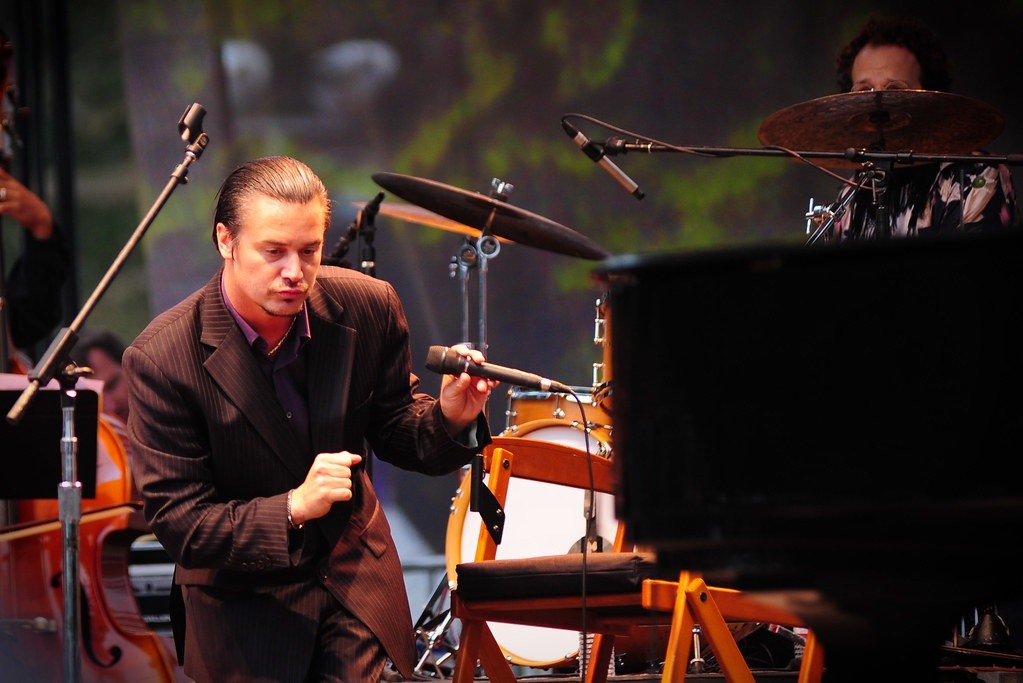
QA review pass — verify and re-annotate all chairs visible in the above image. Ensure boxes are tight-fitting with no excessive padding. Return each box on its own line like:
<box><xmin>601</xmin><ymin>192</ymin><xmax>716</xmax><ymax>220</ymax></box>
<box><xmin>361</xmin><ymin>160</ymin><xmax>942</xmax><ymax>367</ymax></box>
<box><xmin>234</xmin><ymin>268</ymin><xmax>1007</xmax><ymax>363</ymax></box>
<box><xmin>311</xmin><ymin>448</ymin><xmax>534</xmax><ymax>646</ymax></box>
<box><xmin>448</xmin><ymin>437</ymin><xmax>825</xmax><ymax>683</ymax></box>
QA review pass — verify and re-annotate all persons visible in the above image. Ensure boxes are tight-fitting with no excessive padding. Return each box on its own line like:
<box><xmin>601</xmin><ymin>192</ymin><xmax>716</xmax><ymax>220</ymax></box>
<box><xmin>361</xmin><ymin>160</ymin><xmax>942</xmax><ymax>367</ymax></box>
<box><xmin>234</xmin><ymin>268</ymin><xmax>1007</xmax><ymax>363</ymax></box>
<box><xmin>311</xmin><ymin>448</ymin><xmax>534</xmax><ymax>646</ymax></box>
<box><xmin>78</xmin><ymin>331</ymin><xmax>127</xmax><ymax>421</ymax></box>
<box><xmin>122</xmin><ymin>155</ymin><xmax>501</xmax><ymax>683</ymax></box>
<box><xmin>1</xmin><ymin>43</ymin><xmax>57</xmax><ymax>375</ymax></box>
<box><xmin>820</xmin><ymin>27</ymin><xmax>1015</xmax><ymax>245</ymax></box>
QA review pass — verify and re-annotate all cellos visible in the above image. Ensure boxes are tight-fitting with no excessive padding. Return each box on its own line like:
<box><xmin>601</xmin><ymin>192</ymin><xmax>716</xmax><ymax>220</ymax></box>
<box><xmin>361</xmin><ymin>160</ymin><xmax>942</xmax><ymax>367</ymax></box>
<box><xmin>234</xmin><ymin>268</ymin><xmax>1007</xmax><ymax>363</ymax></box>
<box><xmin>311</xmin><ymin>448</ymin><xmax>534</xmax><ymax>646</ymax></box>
<box><xmin>0</xmin><ymin>210</ymin><xmax>191</xmax><ymax>683</ymax></box>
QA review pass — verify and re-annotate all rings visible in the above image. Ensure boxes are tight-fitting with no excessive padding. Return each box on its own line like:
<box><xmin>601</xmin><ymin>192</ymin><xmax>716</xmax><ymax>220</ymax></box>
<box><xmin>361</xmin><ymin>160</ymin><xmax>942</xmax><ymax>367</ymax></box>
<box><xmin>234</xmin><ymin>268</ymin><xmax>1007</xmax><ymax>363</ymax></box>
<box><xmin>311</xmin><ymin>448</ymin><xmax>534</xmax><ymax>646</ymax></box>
<box><xmin>1</xmin><ymin>188</ymin><xmax>8</xmax><ymax>200</ymax></box>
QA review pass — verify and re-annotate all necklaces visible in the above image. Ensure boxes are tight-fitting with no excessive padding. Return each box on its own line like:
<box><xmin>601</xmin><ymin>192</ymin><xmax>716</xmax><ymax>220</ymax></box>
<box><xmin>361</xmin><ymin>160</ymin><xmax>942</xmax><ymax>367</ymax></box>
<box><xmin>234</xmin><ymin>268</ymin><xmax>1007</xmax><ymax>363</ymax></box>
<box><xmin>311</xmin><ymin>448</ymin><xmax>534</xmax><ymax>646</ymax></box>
<box><xmin>267</xmin><ymin>316</ymin><xmax>296</xmax><ymax>356</ymax></box>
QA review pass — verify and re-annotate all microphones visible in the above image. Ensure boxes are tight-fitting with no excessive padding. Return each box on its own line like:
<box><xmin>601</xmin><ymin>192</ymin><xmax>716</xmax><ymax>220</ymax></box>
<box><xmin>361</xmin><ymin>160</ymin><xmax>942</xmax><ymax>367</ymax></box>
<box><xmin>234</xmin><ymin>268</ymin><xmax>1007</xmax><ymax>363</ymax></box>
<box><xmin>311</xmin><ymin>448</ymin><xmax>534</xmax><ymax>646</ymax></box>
<box><xmin>326</xmin><ymin>192</ymin><xmax>386</xmax><ymax>266</ymax></box>
<box><xmin>561</xmin><ymin>119</ymin><xmax>644</xmax><ymax>200</ymax></box>
<box><xmin>425</xmin><ymin>345</ymin><xmax>572</xmax><ymax>394</ymax></box>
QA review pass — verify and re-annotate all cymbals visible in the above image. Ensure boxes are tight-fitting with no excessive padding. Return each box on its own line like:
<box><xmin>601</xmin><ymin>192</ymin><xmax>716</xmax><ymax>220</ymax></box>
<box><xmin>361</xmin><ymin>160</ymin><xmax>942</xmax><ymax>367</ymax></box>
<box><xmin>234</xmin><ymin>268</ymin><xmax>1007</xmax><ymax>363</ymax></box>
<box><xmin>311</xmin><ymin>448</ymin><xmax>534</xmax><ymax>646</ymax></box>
<box><xmin>353</xmin><ymin>198</ymin><xmax>514</xmax><ymax>247</ymax></box>
<box><xmin>370</xmin><ymin>169</ymin><xmax>610</xmax><ymax>264</ymax></box>
<box><xmin>755</xmin><ymin>88</ymin><xmax>1006</xmax><ymax>175</ymax></box>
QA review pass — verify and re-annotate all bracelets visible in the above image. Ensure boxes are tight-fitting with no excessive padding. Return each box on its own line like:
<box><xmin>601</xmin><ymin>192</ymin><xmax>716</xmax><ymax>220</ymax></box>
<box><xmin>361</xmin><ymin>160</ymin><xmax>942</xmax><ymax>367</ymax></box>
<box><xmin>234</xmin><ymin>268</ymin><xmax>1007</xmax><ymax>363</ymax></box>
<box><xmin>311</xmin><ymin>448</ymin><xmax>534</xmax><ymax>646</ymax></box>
<box><xmin>287</xmin><ymin>489</ymin><xmax>305</xmax><ymax>530</ymax></box>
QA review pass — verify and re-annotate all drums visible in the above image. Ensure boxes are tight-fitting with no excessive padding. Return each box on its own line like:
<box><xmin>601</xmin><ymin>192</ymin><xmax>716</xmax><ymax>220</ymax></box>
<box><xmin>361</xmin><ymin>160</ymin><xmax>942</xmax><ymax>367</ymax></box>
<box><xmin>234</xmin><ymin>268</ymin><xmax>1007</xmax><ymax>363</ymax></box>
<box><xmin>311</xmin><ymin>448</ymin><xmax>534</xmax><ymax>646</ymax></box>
<box><xmin>440</xmin><ymin>417</ymin><xmax>634</xmax><ymax>671</ymax></box>
<box><xmin>501</xmin><ymin>386</ymin><xmax>613</xmax><ymax>439</ymax></box>
